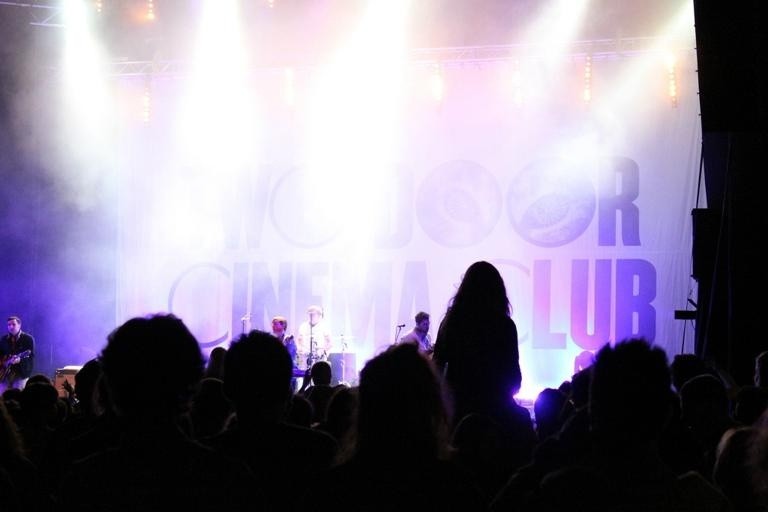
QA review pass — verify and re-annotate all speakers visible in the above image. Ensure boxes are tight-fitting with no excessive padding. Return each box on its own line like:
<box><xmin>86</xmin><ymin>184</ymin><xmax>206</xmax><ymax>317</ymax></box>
<box><xmin>54</xmin><ymin>369</ymin><xmax>78</xmax><ymax>398</ymax></box>
<box><xmin>327</xmin><ymin>353</ymin><xmax>357</xmax><ymax>385</ymax></box>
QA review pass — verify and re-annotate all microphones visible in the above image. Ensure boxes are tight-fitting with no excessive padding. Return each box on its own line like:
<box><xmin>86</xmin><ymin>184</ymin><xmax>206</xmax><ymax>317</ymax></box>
<box><xmin>397</xmin><ymin>324</ymin><xmax>405</xmax><ymax>327</ymax></box>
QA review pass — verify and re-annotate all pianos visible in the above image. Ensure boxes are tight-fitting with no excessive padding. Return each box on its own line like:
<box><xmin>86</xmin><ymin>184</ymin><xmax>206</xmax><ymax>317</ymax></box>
<box><xmin>291</xmin><ymin>367</ymin><xmax>311</xmax><ymax>378</ymax></box>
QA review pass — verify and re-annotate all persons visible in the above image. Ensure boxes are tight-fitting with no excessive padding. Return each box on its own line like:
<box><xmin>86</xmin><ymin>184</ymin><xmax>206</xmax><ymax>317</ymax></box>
<box><xmin>433</xmin><ymin>261</ymin><xmax>522</xmax><ymax>417</ymax></box>
<box><xmin>0</xmin><ymin>312</ymin><xmax>767</xmax><ymax>512</ymax></box>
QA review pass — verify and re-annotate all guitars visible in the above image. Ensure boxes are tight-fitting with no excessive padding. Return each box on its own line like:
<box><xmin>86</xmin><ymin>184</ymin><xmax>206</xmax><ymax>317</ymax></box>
<box><xmin>0</xmin><ymin>349</ymin><xmax>31</xmax><ymax>381</ymax></box>
